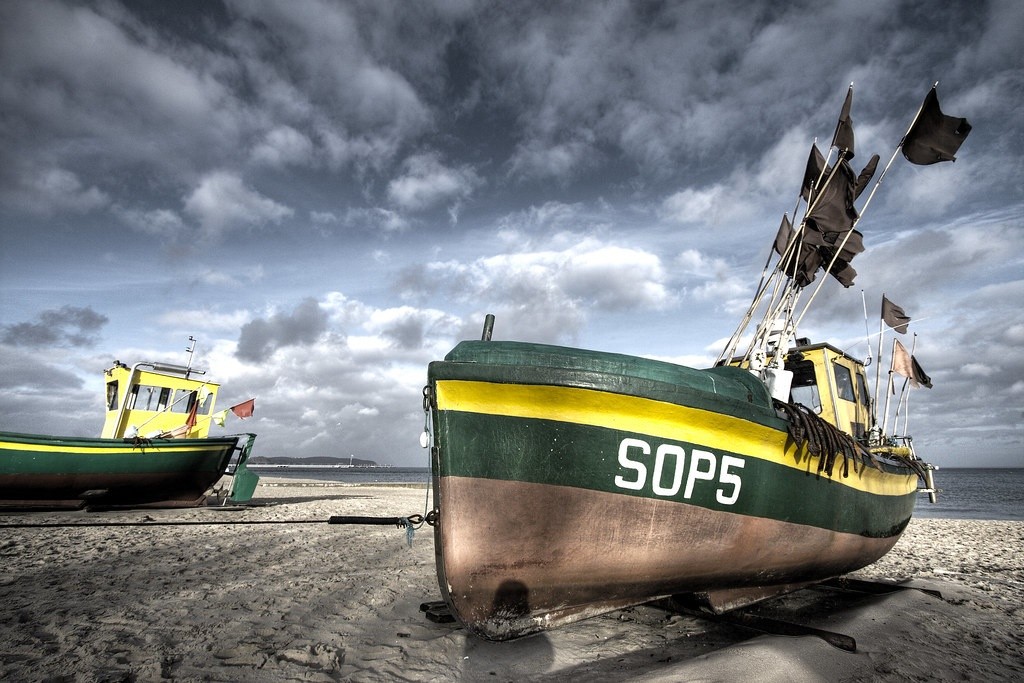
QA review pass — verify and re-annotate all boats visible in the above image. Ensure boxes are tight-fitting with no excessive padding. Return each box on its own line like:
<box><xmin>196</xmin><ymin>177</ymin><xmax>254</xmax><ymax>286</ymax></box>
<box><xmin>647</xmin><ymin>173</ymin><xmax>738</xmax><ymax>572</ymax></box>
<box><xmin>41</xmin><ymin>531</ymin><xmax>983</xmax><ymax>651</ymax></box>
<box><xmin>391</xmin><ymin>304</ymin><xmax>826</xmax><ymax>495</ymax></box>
<box><xmin>422</xmin><ymin>290</ymin><xmax>939</xmax><ymax>643</ymax></box>
<box><xmin>0</xmin><ymin>336</ymin><xmax>260</xmax><ymax>511</ymax></box>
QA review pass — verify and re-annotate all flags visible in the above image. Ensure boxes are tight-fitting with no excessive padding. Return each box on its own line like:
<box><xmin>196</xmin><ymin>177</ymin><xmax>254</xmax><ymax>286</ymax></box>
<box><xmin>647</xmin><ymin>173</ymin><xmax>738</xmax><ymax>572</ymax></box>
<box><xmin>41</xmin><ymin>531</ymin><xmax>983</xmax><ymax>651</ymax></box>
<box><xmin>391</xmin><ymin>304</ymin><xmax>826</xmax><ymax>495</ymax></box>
<box><xmin>898</xmin><ymin>87</ymin><xmax>973</xmax><ymax>165</ymax></box>
<box><xmin>774</xmin><ymin>215</ymin><xmax>796</xmax><ymax>257</ymax></box>
<box><xmin>911</xmin><ymin>355</ymin><xmax>932</xmax><ymax>388</ymax></box>
<box><xmin>881</xmin><ymin>298</ymin><xmax>911</xmax><ymax>334</ymax></box>
<box><xmin>196</xmin><ymin>385</ymin><xmax>210</xmax><ymax>407</ymax></box>
<box><xmin>212</xmin><ymin>410</ymin><xmax>230</xmax><ymax>427</ymax></box>
<box><xmin>780</xmin><ymin>88</ymin><xmax>880</xmax><ymax>288</ymax></box>
<box><xmin>231</xmin><ymin>398</ymin><xmax>254</xmax><ymax>418</ymax></box>
<box><xmin>185</xmin><ymin>399</ymin><xmax>199</xmax><ymax>433</ymax></box>
<box><xmin>892</xmin><ymin>340</ymin><xmax>919</xmax><ymax>389</ymax></box>
